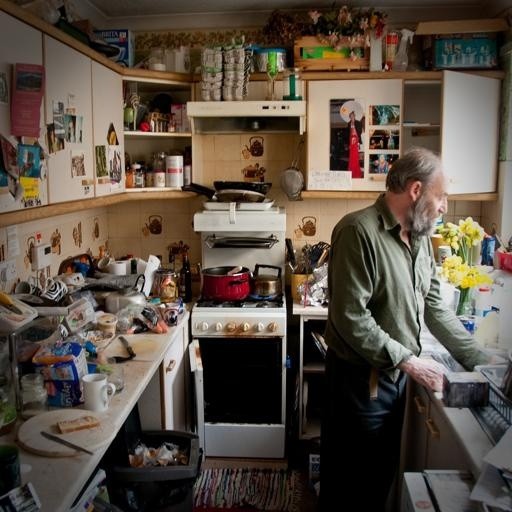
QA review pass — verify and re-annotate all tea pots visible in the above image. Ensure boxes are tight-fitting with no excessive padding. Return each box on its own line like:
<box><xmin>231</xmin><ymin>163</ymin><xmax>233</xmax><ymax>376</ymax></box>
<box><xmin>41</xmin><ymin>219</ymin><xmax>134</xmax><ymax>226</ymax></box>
<box><xmin>24</xmin><ymin>234</ymin><xmax>38</xmax><ymax>262</ymax></box>
<box><xmin>92</xmin><ymin>217</ymin><xmax>100</xmax><ymax>238</ymax></box>
<box><xmin>297</xmin><ymin>215</ymin><xmax>317</xmax><ymax>237</ymax></box>
<box><xmin>144</xmin><ymin>214</ymin><xmax>164</xmax><ymax>235</ymax></box>
<box><xmin>245</xmin><ymin>135</ymin><xmax>265</xmax><ymax>157</ymax></box>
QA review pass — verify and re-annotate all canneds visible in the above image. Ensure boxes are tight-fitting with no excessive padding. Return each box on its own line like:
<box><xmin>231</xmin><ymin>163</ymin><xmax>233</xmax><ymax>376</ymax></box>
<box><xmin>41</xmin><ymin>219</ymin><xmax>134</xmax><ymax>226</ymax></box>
<box><xmin>166</xmin><ymin>155</ymin><xmax>184</xmax><ymax>187</ymax></box>
<box><xmin>133</xmin><ymin>169</ymin><xmax>146</xmax><ymax>187</ymax></box>
<box><xmin>152</xmin><ymin>269</ymin><xmax>177</xmax><ymax>303</ymax></box>
<box><xmin>0</xmin><ymin>376</ymin><xmax>18</xmax><ymax>434</ymax></box>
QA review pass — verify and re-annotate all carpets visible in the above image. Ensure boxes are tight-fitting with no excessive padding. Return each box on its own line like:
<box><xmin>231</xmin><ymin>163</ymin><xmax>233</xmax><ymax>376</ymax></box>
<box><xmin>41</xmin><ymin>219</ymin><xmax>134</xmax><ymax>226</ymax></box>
<box><xmin>191</xmin><ymin>465</ymin><xmax>303</xmax><ymax>511</ymax></box>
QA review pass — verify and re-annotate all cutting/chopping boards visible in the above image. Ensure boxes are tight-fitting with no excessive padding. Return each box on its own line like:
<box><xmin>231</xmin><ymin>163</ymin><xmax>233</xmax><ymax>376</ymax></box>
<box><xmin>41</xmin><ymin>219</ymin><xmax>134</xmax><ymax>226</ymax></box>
<box><xmin>102</xmin><ymin>333</ymin><xmax>166</xmax><ymax>362</ymax></box>
<box><xmin>16</xmin><ymin>407</ymin><xmax>117</xmax><ymax>456</ymax></box>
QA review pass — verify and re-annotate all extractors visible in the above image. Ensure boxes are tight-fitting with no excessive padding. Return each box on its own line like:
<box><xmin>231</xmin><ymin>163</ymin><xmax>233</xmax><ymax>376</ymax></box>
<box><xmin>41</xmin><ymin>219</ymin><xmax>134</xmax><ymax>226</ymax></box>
<box><xmin>186</xmin><ymin>99</ymin><xmax>305</xmax><ymax>135</ymax></box>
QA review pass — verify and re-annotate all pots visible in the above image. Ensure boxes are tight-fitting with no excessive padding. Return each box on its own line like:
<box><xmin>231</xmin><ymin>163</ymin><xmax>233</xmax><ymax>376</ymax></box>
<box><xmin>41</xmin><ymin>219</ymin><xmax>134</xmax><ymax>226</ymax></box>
<box><xmin>177</xmin><ymin>178</ymin><xmax>271</xmax><ymax>202</ymax></box>
<box><xmin>201</xmin><ymin>263</ymin><xmax>283</xmax><ymax>302</ymax></box>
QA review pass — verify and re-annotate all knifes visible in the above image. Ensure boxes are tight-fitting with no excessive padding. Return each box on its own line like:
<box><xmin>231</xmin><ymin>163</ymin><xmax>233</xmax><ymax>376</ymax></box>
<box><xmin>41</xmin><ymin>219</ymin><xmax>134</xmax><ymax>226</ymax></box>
<box><xmin>41</xmin><ymin>429</ymin><xmax>95</xmax><ymax>456</ymax></box>
<box><xmin>119</xmin><ymin>335</ymin><xmax>137</xmax><ymax>358</ymax></box>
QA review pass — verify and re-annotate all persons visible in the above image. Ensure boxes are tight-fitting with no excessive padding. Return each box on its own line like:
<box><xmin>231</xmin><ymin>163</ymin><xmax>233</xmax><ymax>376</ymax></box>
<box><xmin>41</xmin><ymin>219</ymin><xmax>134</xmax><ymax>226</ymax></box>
<box><xmin>341</xmin><ymin>111</ymin><xmax>363</xmax><ymax>178</ymax></box>
<box><xmin>318</xmin><ymin>145</ymin><xmax>503</xmax><ymax>512</ymax></box>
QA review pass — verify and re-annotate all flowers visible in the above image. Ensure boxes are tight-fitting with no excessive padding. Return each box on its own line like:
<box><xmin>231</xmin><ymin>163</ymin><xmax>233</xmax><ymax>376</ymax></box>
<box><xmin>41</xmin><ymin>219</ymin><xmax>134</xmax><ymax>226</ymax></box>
<box><xmin>434</xmin><ymin>214</ymin><xmax>485</xmax><ymax>266</ymax></box>
<box><xmin>438</xmin><ymin>253</ymin><xmax>495</xmax><ymax>315</ymax></box>
<box><xmin>306</xmin><ymin>0</ymin><xmax>389</xmax><ymax>63</ymax></box>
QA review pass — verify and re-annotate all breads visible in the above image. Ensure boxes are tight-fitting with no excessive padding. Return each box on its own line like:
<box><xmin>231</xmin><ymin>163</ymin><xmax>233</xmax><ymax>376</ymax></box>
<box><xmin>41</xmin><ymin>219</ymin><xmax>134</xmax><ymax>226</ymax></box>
<box><xmin>56</xmin><ymin>415</ymin><xmax>99</xmax><ymax>435</ymax></box>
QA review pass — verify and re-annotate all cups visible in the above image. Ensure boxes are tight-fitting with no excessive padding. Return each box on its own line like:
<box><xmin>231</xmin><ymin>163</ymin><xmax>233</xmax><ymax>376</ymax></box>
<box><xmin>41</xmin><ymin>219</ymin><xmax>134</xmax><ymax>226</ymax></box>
<box><xmin>82</xmin><ymin>374</ymin><xmax>117</xmax><ymax>411</ymax></box>
<box><xmin>437</xmin><ymin>246</ymin><xmax>453</xmax><ymax>266</ymax></box>
<box><xmin>291</xmin><ymin>273</ymin><xmax>314</xmax><ymax>300</ymax></box>
<box><xmin>14</xmin><ymin>280</ymin><xmax>32</xmax><ymax>296</ymax></box>
<box><xmin>40</xmin><ymin>277</ymin><xmax>68</xmax><ymax>302</ymax></box>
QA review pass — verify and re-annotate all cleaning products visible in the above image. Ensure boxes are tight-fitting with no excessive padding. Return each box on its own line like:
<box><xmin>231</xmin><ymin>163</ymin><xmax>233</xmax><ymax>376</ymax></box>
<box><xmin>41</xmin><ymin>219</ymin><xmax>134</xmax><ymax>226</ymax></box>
<box><xmin>391</xmin><ymin>28</ymin><xmax>415</xmax><ymax>73</ymax></box>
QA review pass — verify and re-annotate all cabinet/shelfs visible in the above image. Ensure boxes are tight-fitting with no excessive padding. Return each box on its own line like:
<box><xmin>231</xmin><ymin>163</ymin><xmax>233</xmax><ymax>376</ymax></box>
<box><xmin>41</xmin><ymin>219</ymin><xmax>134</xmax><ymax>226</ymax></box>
<box><xmin>407</xmin><ymin>380</ymin><xmax>470</xmax><ymax>472</ymax></box>
<box><xmin>297</xmin><ymin>68</ymin><xmax>506</xmax><ymax>202</ymax></box>
<box><xmin>92</xmin><ymin>46</ymin><xmax>122</xmax><ymax>208</ymax></box>
<box><xmin>288</xmin><ymin>300</ymin><xmax>329</xmax><ymax>442</ymax></box>
<box><xmin>1</xmin><ymin>8</ymin><xmax>49</xmax><ymax>231</ymax></box>
<box><xmin>122</xmin><ymin>63</ymin><xmax>196</xmax><ymax>207</ymax></box>
<box><xmin>136</xmin><ymin>311</ymin><xmax>195</xmax><ymax>434</ymax></box>
<box><xmin>43</xmin><ymin>16</ymin><xmax>93</xmax><ymax>220</ymax></box>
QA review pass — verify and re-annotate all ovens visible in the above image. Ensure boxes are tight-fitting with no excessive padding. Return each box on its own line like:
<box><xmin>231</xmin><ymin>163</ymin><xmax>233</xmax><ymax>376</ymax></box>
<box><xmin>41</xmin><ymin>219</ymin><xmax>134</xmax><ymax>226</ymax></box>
<box><xmin>188</xmin><ymin>334</ymin><xmax>287</xmax><ymax>464</ymax></box>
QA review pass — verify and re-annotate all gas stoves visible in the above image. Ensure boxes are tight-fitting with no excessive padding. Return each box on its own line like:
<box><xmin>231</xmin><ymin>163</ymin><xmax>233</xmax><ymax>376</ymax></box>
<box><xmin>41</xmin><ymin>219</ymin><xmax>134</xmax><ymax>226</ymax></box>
<box><xmin>190</xmin><ymin>295</ymin><xmax>285</xmax><ymax>338</ymax></box>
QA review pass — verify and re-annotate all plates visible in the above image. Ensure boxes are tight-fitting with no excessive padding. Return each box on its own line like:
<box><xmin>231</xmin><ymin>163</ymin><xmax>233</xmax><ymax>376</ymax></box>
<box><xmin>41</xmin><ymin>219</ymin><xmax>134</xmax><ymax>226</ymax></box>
<box><xmin>72</xmin><ymin>330</ymin><xmax>114</xmax><ymax>346</ymax></box>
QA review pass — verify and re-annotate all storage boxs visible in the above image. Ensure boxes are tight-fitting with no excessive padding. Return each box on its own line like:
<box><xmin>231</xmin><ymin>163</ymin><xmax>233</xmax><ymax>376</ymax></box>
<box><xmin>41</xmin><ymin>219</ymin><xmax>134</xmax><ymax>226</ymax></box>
<box><xmin>409</xmin><ymin>15</ymin><xmax>510</xmax><ymax>73</ymax></box>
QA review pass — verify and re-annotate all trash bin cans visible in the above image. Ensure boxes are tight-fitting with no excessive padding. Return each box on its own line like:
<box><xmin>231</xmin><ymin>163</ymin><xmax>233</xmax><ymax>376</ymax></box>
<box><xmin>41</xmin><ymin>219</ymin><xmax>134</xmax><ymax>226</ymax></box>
<box><xmin>100</xmin><ymin>430</ymin><xmax>203</xmax><ymax>512</ymax></box>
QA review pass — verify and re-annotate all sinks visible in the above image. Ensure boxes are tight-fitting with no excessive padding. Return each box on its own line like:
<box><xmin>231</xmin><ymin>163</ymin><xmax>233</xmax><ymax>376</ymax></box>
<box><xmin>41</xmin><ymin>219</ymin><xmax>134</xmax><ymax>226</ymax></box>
<box><xmin>431</xmin><ymin>348</ymin><xmax>512</xmax><ymax>447</ymax></box>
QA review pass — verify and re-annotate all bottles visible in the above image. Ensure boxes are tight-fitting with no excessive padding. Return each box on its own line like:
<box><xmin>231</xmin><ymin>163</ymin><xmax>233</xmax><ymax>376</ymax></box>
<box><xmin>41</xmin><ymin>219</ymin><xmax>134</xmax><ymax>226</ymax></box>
<box><xmin>481</xmin><ymin>237</ymin><xmax>496</xmax><ymax>265</ymax></box>
<box><xmin>19</xmin><ymin>372</ymin><xmax>48</xmax><ymax>419</ymax></box>
<box><xmin>283</xmin><ymin>67</ymin><xmax>303</xmax><ymax>99</ymax></box>
<box><xmin>125</xmin><ymin>162</ymin><xmax>165</xmax><ymax>188</ymax></box>
<box><xmin>148</xmin><ymin>46</ymin><xmax>166</xmax><ymax>72</ymax></box>
<box><xmin>106</xmin><ymin>283</ymin><xmax>148</xmax><ymax>314</ymax></box>
<box><xmin>179</xmin><ymin>250</ymin><xmax>192</xmax><ymax>303</ymax></box>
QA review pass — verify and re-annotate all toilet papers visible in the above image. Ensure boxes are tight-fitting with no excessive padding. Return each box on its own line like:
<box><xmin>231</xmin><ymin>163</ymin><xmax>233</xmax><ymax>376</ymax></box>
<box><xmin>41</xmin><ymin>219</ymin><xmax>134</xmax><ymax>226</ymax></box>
<box><xmin>109</xmin><ymin>260</ymin><xmax>126</xmax><ymax>276</ymax></box>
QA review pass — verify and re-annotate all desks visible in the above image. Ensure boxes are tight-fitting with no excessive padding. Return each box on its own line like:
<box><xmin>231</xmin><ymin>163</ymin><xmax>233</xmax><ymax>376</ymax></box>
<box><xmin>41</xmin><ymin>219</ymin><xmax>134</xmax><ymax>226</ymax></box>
<box><xmin>0</xmin><ymin>336</ymin><xmax>163</xmax><ymax>511</ymax></box>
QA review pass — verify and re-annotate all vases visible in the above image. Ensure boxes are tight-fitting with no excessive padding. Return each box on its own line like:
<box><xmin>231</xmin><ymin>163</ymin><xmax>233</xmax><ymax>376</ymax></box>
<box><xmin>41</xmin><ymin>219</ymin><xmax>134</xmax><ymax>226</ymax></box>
<box><xmin>454</xmin><ymin>286</ymin><xmax>471</xmax><ymax>318</ymax></box>
